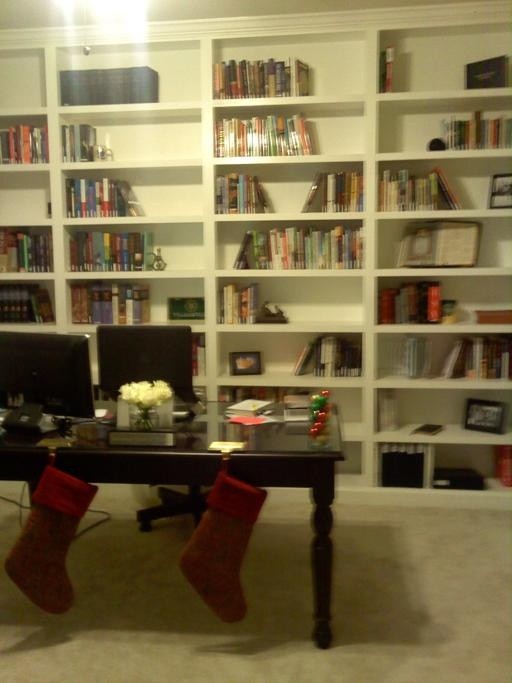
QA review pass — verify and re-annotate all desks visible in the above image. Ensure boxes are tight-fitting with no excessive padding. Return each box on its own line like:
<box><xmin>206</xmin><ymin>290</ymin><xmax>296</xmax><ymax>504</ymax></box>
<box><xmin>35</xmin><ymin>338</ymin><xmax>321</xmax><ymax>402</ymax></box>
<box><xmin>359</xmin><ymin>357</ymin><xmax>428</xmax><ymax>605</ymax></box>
<box><xmin>0</xmin><ymin>402</ymin><xmax>346</xmax><ymax>650</ymax></box>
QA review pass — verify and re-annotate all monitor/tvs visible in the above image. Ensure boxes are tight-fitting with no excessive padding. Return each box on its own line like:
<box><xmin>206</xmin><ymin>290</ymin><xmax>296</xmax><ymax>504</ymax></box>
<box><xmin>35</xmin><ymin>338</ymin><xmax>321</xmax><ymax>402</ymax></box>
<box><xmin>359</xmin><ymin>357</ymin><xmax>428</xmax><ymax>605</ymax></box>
<box><xmin>0</xmin><ymin>331</ymin><xmax>94</xmax><ymax>436</ymax></box>
<box><xmin>96</xmin><ymin>324</ymin><xmax>199</xmax><ymax>403</ymax></box>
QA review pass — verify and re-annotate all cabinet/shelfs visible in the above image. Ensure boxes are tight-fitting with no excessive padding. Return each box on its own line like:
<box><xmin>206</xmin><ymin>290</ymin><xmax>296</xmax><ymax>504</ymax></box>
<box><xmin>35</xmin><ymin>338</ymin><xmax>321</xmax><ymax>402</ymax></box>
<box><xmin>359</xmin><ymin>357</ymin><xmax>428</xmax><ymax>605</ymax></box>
<box><xmin>378</xmin><ymin>87</ymin><xmax>512</xmax><ymax>443</ymax></box>
<box><xmin>0</xmin><ymin>107</ymin><xmax>56</xmax><ymax>334</ymax></box>
<box><xmin>58</xmin><ymin>99</ymin><xmax>202</xmax><ymax>387</ymax></box>
<box><xmin>212</xmin><ymin>92</ymin><xmax>365</xmax><ymax>442</ymax></box>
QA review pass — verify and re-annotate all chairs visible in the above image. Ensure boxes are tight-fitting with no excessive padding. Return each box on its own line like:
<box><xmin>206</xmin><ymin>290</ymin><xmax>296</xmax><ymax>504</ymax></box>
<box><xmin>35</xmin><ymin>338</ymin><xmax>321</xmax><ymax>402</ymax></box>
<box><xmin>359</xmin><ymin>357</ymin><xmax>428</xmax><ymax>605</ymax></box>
<box><xmin>97</xmin><ymin>324</ymin><xmax>211</xmax><ymax>532</ymax></box>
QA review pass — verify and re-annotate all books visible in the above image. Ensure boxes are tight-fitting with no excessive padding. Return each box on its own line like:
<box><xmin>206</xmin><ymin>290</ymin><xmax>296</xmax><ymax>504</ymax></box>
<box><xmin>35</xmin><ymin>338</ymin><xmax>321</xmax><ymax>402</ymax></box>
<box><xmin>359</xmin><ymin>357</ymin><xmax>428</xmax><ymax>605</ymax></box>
<box><xmin>0</xmin><ymin>123</ymin><xmax>150</xmax><ymax>325</ymax></box>
<box><xmin>218</xmin><ymin>385</ymin><xmax>298</xmax><ymax>418</ymax></box>
<box><xmin>218</xmin><ymin>283</ymin><xmax>258</xmax><ymax>324</ymax></box>
<box><xmin>234</xmin><ymin>225</ymin><xmax>363</xmax><ymax>270</ymax></box>
<box><xmin>216</xmin><ymin>113</ymin><xmax>313</xmax><ymax>157</ymax></box>
<box><xmin>191</xmin><ymin>335</ymin><xmax>207</xmax><ymax>377</ymax></box>
<box><xmin>380</xmin><ymin>166</ymin><xmax>462</xmax><ymax>212</ymax></box>
<box><xmin>302</xmin><ymin>169</ymin><xmax>363</xmax><ymax>213</ymax></box>
<box><xmin>378</xmin><ymin>390</ymin><xmax>442</xmax><ymax>434</ymax></box>
<box><xmin>398</xmin><ymin>220</ymin><xmax>483</xmax><ymax>268</ymax></box>
<box><xmin>214</xmin><ymin>54</ymin><xmax>309</xmax><ymax>98</ymax></box>
<box><xmin>294</xmin><ymin>336</ymin><xmax>361</xmax><ymax>376</ymax></box>
<box><xmin>405</xmin><ymin>335</ymin><xmax>510</xmax><ymax>379</ymax></box>
<box><xmin>216</xmin><ymin>173</ymin><xmax>270</xmax><ymax>214</ymax></box>
<box><xmin>378</xmin><ymin>281</ymin><xmax>512</xmax><ymax>325</ymax></box>
<box><xmin>380</xmin><ymin>48</ymin><xmax>510</xmax><ymax>150</ymax></box>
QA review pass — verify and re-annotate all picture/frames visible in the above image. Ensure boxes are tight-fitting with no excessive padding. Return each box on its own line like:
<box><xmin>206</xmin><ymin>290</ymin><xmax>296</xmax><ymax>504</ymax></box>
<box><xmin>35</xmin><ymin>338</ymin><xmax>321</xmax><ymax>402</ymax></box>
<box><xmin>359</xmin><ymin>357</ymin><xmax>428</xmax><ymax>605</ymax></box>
<box><xmin>489</xmin><ymin>173</ymin><xmax>512</xmax><ymax>208</ymax></box>
<box><xmin>465</xmin><ymin>398</ymin><xmax>505</xmax><ymax>434</ymax></box>
